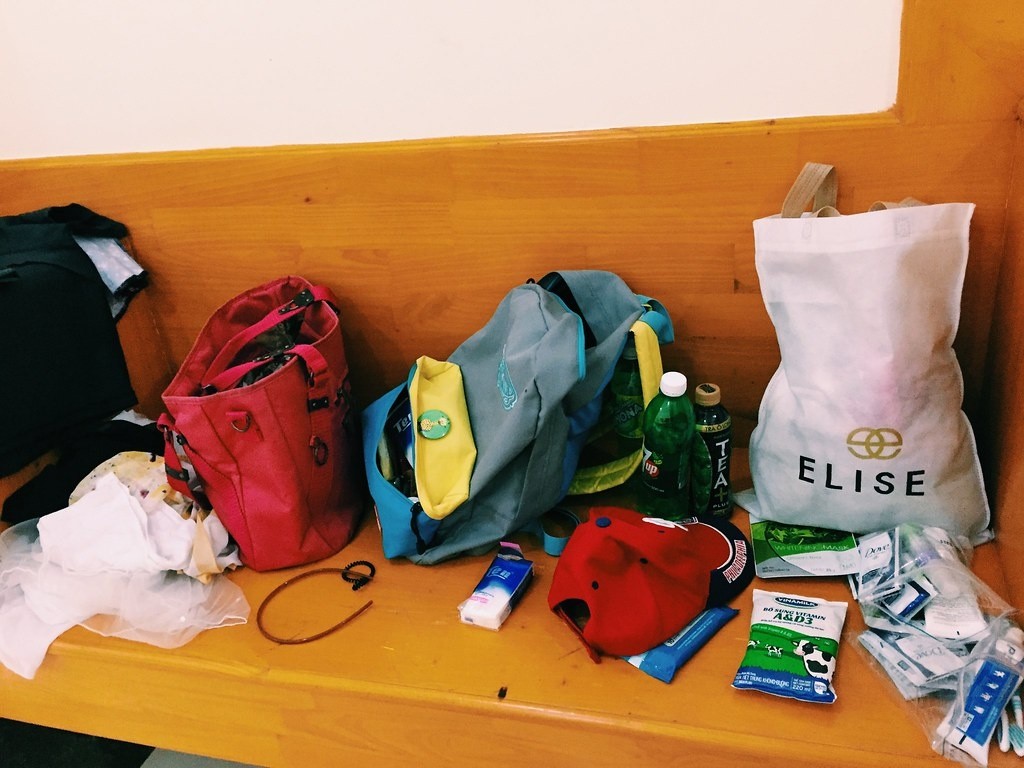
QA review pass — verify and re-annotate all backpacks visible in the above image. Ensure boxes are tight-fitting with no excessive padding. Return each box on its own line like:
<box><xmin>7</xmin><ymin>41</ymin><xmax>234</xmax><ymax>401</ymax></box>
<box><xmin>358</xmin><ymin>267</ymin><xmax>675</xmax><ymax>565</ymax></box>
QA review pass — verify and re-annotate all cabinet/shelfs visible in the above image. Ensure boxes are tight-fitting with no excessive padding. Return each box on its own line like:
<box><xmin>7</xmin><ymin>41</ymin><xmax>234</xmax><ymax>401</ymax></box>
<box><xmin>1</xmin><ymin>0</ymin><xmax>1024</xmax><ymax>768</ymax></box>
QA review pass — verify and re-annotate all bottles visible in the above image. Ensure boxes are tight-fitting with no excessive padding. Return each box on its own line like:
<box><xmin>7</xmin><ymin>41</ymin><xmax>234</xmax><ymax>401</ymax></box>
<box><xmin>693</xmin><ymin>382</ymin><xmax>733</xmax><ymax>520</ymax></box>
<box><xmin>631</xmin><ymin>371</ymin><xmax>692</xmax><ymax>521</ymax></box>
<box><xmin>612</xmin><ymin>328</ymin><xmax>646</xmax><ymax>458</ymax></box>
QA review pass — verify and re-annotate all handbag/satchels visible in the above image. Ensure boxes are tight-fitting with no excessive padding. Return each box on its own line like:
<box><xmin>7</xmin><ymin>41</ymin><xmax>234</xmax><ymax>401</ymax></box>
<box><xmin>157</xmin><ymin>276</ymin><xmax>365</xmax><ymax>575</ymax></box>
<box><xmin>734</xmin><ymin>160</ymin><xmax>997</xmax><ymax>553</ymax></box>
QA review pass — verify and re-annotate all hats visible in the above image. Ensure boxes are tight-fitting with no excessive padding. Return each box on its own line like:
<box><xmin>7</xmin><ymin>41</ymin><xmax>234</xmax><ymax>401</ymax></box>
<box><xmin>550</xmin><ymin>507</ymin><xmax>755</xmax><ymax>662</ymax></box>
